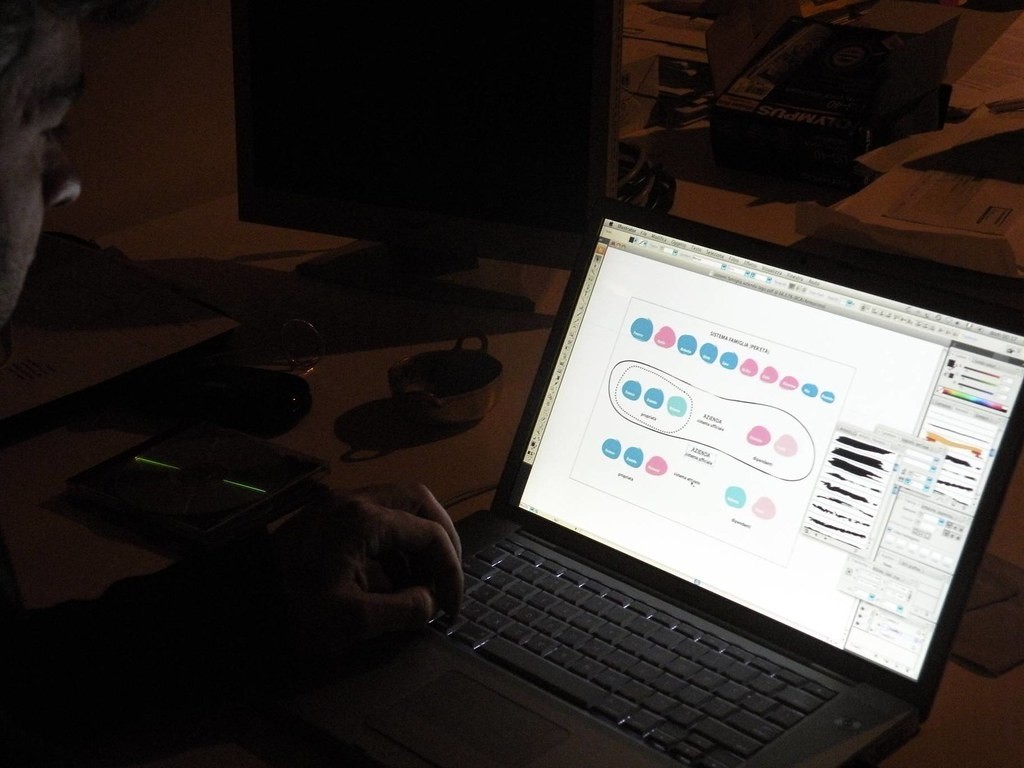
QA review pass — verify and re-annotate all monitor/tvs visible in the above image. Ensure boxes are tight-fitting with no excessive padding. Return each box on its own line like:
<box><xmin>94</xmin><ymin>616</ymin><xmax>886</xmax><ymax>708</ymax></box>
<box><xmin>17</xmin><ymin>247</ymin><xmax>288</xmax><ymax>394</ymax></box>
<box><xmin>226</xmin><ymin>0</ymin><xmax>623</xmax><ymax>309</ymax></box>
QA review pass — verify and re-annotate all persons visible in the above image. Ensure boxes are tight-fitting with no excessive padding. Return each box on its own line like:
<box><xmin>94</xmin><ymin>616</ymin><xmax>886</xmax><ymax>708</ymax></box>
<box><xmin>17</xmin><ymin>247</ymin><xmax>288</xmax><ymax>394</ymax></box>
<box><xmin>0</xmin><ymin>0</ymin><xmax>465</xmax><ymax>768</ymax></box>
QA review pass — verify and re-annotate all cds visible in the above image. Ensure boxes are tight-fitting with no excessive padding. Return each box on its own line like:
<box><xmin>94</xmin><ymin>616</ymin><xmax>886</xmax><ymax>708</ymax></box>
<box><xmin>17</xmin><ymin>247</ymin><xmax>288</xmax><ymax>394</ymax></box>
<box><xmin>114</xmin><ymin>435</ymin><xmax>290</xmax><ymax>515</ymax></box>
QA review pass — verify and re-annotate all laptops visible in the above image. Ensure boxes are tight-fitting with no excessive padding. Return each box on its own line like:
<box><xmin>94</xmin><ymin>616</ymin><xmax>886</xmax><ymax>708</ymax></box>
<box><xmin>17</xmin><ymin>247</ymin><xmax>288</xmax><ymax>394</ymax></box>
<box><xmin>259</xmin><ymin>195</ymin><xmax>1024</xmax><ymax>768</ymax></box>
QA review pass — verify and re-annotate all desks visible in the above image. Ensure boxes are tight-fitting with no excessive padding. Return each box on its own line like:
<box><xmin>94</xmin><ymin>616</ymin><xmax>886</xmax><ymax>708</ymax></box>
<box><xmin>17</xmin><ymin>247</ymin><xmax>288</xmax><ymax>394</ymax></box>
<box><xmin>0</xmin><ymin>178</ymin><xmax>1023</xmax><ymax>768</ymax></box>
